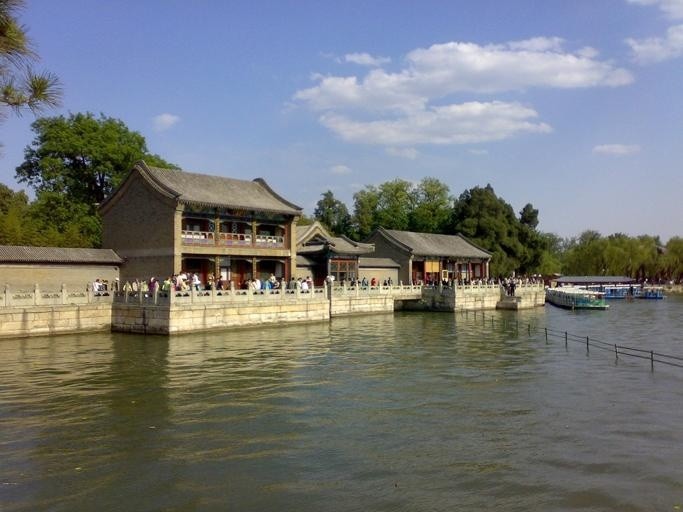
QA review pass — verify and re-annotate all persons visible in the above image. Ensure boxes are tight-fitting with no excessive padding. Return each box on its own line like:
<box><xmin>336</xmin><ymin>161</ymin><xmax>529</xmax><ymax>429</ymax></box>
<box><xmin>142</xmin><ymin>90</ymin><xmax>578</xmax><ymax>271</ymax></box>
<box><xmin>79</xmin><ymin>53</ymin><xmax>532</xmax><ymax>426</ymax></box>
<box><xmin>240</xmin><ymin>272</ymin><xmax>312</xmax><ymax>294</ymax></box>
<box><xmin>326</xmin><ymin>274</ymin><xmax>393</xmax><ymax>290</ymax></box>
<box><xmin>89</xmin><ymin>270</ymin><xmax>225</xmax><ymax>298</ymax></box>
<box><xmin>516</xmin><ymin>276</ymin><xmax>535</xmax><ymax>284</ymax></box>
<box><xmin>403</xmin><ymin>276</ymin><xmax>516</xmax><ymax>297</ymax></box>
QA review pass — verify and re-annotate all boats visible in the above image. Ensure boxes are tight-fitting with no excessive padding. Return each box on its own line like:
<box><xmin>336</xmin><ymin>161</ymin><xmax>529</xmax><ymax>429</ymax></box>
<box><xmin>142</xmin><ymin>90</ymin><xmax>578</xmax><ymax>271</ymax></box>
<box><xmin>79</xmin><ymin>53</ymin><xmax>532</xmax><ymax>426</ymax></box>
<box><xmin>544</xmin><ymin>287</ymin><xmax>611</xmax><ymax>310</ymax></box>
<box><xmin>586</xmin><ymin>284</ymin><xmax>667</xmax><ymax>300</ymax></box>
